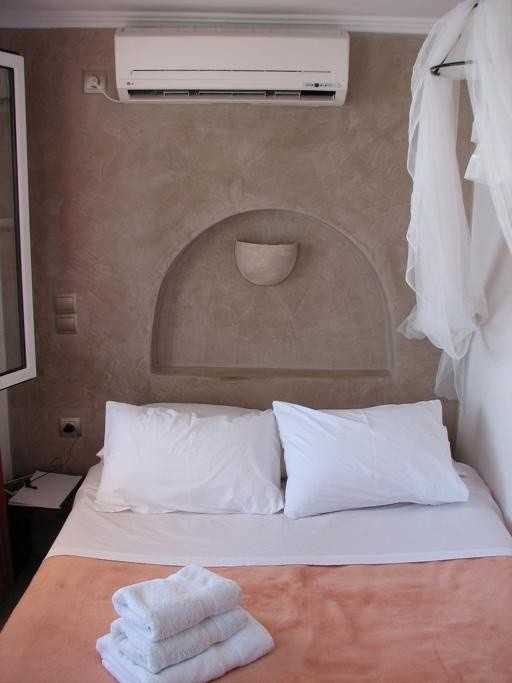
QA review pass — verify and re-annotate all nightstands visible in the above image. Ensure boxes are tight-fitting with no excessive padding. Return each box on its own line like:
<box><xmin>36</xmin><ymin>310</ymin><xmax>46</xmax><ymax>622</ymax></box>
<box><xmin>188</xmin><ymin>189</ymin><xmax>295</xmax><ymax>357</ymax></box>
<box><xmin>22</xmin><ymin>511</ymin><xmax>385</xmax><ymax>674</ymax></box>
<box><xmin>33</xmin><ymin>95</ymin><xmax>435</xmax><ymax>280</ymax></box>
<box><xmin>6</xmin><ymin>470</ymin><xmax>82</xmax><ymax>575</ymax></box>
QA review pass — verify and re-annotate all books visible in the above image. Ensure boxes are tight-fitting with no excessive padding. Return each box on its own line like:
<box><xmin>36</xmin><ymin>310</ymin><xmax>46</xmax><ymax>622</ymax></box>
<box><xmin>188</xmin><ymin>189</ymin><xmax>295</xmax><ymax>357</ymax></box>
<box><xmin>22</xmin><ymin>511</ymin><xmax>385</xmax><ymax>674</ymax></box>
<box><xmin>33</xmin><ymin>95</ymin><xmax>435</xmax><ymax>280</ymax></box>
<box><xmin>10</xmin><ymin>470</ymin><xmax>85</xmax><ymax>511</ymax></box>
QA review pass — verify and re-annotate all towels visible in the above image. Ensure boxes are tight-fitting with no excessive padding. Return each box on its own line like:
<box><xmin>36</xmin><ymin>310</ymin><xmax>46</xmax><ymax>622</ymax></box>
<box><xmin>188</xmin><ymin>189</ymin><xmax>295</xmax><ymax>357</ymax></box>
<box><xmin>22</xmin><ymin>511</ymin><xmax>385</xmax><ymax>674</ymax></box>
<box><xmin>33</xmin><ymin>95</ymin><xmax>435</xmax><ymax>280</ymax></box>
<box><xmin>92</xmin><ymin>609</ymin><xmax>278</xmax><ymax>683</ymax></box>
<box><xmin>112</xmin><ymin>562</ymin><xmax>244</xmax><ymax>643</ymax></box>
<box><xmin>105</xmin><ymin>608</ymin><xmax>251</xmax><ymax>675</ymax></box>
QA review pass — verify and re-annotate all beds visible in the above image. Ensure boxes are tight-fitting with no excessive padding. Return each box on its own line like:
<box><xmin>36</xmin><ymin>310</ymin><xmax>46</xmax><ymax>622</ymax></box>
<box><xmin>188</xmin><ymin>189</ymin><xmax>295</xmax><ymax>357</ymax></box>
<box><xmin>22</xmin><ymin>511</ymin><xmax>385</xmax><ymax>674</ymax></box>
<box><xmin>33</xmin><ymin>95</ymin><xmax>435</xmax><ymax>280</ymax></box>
<box><xmin>0</xmin><ymin>403</ymin><xmax>512</xmax><ymax>683</ymax></box>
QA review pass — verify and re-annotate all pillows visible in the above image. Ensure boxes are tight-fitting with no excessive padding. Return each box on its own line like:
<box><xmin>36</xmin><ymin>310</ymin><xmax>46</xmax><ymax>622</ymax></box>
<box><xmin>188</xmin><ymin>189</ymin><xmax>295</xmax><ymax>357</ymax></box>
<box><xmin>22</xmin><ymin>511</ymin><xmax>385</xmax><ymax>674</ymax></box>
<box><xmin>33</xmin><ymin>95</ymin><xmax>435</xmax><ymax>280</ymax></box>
<box><xmin>272</xmin><ymin>393</ymin><xmax>476</xmax><ymax>521</ymax></box>
<box><xmin>92</xmin><ymin>397</ymin><xmax>288</xmax><ymax>516</ymax></box>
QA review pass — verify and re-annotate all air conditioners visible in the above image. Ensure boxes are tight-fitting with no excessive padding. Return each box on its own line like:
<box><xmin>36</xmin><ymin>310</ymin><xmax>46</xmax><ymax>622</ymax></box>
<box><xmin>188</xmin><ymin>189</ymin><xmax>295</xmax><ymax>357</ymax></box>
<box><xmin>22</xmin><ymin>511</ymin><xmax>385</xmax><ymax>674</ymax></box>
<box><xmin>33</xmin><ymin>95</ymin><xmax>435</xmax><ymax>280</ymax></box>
<box><xmin>111</xmin><ymin>27</ymin><xmax>352</xmax><ymax>114</ymax></box>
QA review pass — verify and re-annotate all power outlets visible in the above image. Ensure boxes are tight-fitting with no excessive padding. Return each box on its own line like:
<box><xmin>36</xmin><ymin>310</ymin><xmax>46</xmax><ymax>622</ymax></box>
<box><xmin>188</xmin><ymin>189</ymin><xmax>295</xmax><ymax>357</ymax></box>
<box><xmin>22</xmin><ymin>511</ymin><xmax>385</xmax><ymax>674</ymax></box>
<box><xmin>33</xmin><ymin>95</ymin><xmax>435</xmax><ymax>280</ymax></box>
<box><xmin>57</xmin><ymin>413</ymin><xmax>86</xmax><ymax>437</ymax></box>
